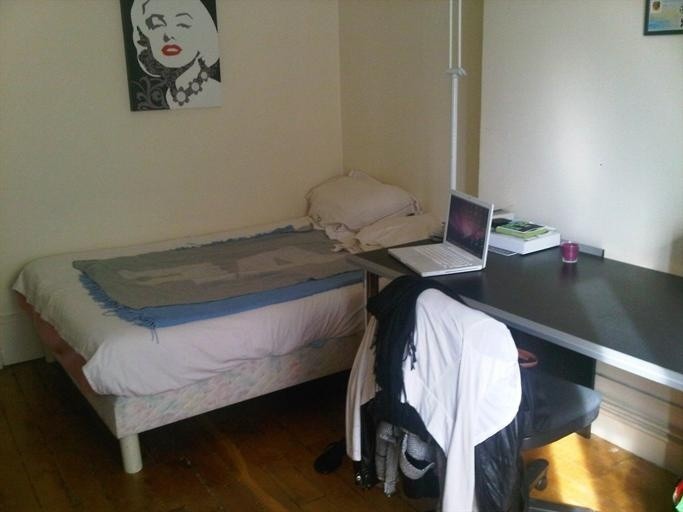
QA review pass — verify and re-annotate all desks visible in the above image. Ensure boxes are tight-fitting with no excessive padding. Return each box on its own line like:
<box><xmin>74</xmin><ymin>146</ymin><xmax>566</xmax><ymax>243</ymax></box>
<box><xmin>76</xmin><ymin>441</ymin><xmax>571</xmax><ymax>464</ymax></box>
<box><xmin>348</xmin><ymin>229</ymin><xmax>681</xmax><ymax>481</ymax></box>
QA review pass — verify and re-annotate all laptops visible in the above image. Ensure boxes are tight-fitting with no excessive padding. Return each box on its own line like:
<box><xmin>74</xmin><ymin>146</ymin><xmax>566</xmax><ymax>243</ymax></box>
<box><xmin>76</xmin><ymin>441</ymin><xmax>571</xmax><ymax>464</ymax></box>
<box><xmin>388</xmin><ymin>189</ymin><xmax>494</xmax><ymax>277</ymax></box>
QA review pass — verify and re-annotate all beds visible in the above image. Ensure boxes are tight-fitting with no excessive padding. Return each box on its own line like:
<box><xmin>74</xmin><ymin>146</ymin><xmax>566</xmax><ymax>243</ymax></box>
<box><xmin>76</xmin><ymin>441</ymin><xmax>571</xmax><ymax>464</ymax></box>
<box><xmin>10</xmin><ymin>216</ymin><xmax>443</xmax><ymax>474</ymax></box>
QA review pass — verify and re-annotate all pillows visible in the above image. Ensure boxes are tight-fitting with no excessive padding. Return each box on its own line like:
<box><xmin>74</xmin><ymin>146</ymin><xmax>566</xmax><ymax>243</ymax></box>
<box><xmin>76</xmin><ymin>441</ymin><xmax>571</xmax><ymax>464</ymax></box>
<box><xmin>303</xmin><ymin>209</ymin><xmax>442</xmax><ymax>253</ymax></box>
<box><xmin>302</xmin><ymin>170</ymin><xmax>420</xmax><ymax>245</ymax></box>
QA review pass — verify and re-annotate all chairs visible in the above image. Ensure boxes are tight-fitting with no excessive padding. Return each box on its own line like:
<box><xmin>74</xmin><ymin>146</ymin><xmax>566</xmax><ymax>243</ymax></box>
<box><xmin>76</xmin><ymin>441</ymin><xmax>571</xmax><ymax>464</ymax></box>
<box><xmin>366</xmin><ymin>277</ymin><xmax>601</xmax><ymax>512</ymax></box>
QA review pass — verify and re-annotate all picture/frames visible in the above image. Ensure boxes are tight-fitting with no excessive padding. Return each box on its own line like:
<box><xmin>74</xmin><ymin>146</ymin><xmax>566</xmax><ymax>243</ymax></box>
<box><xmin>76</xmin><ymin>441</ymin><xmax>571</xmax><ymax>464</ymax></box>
<box><xmin>643</xmin><ymin>0</ymin><xmax>683</xmax><ymax>35</ymax></box>
